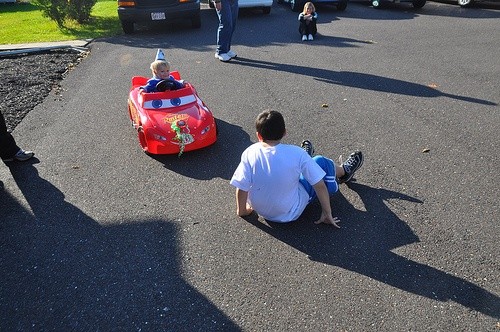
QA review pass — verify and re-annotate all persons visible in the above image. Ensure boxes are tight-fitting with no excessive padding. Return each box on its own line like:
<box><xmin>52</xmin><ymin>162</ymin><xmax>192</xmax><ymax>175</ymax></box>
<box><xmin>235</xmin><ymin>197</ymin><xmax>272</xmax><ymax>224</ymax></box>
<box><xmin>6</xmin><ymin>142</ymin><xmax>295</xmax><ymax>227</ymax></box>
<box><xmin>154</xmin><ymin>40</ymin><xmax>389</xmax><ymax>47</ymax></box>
<box><xmin>210</xmin><ymin>0</ymin><xmax>239</xmax><ymax>62</ymax></box>
<box><xmin>0</xmin><ymin>109</ymin><xmax>35</xmax><ymax>162</ymax></box>
<box><xmin>139</xmin><ymin>60</ymin><xmax>185</xmax><ymax>95</ymax></box>
<box><xmin>297</xmin><ymin>2</ymin><xmax>319</xmax><ymax>41</ymax></box>
<box><xmin>229</xmin><ymin>109</ymin><xmax>364</xmax><ymax>229</ymax></box>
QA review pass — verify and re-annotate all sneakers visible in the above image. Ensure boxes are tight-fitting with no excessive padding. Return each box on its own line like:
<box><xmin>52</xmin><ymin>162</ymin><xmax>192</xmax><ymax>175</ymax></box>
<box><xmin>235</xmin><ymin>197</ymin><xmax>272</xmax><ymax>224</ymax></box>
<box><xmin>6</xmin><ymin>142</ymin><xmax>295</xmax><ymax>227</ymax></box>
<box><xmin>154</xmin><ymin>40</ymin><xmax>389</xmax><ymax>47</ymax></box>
<box><xmin>214</xmin><ymin>51</ymin><xmax>237</xmax><ymax>61</ymax></box>
<box><xmin>301</xmin><ymin>139</ymin><xmax>315</xmax><ymax>156</ymax></box>
<box><xmin>302</xmin><ymin>34</ymin><xmax>307</xmax><ymax>41</ymax></box>
<box><xmin>308</xmin><ymin>34</ymin><xmax>313</xmax><ymax>41</ymax></box>
<box><xmin>340</xmin><ymin>149</ymin><xmax>364</xmax><ymax>182</ymax></box>
<box><xmin>2</xmin><ymin>148</ymin><xmax>35</xmax><ymax>162</ymax></box>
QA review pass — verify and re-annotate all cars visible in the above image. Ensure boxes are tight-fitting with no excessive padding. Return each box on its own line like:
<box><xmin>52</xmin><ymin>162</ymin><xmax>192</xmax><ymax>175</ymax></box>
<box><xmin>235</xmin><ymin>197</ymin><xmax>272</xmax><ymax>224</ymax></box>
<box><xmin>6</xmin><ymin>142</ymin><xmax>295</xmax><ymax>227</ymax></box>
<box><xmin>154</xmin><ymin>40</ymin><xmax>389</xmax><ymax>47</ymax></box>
<box><xmin>363</xmin><ymin>0</ymin><xmax>427</xmax><ymax>11</ymax></box>
<box><xmin>128</xmin><ymin>71</ymin><xmax>218</xmax><ymax>158</ymax></box>
<box><xmin>208</xmin><ymin>0</ymin><xmax>274</xmax><ymax>15</ymax></box>
<box><xmin>279</xmin><ymin>0</ymin><xmax>348</xmax><ymax>12</ymax></box>
<box><xmin>119</xmin><ymin>0</ymin><xmax>201</xmax><ymax>34</ymax></box>
